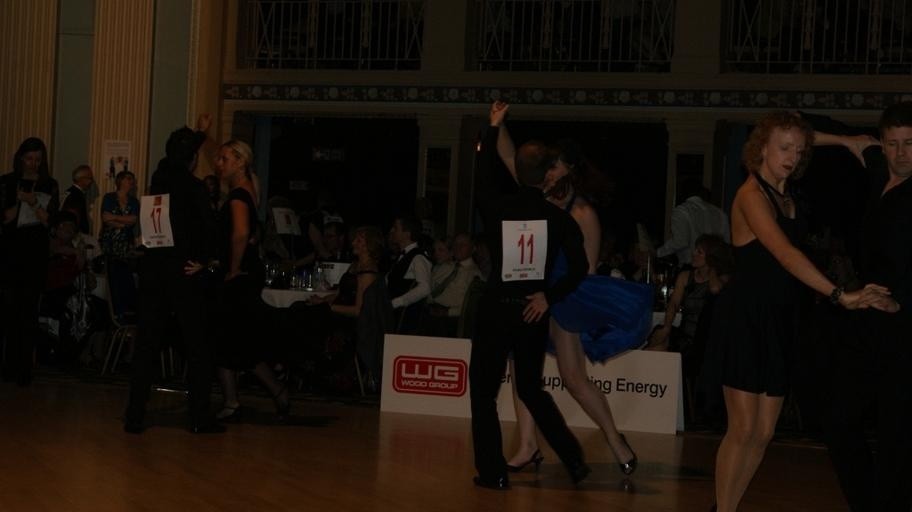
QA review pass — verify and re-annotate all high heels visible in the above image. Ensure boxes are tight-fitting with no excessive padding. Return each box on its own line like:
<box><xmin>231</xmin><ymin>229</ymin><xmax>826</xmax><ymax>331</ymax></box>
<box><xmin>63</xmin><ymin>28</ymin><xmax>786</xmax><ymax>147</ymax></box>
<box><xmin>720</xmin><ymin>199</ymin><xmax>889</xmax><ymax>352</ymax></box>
<box><xmin>505</xmin><ymin>450</ymin><xmax>544</xmax><ymax>472</ymax></box>
<box><xmin>619</xmin><ymin>433</ymin><xmax>637</xmax><ymax>475</ymax></box>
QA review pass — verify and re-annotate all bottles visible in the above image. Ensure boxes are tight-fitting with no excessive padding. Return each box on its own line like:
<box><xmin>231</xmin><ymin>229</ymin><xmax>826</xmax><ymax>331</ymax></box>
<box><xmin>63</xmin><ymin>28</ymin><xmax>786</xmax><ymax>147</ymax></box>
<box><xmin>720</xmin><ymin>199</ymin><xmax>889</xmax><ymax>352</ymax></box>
<box><xmin>656</xmin><ymin>269</ymin><xmax>673</xmax><ymax>304</ymax></box>
<box><xmin>290</xmin><ymin>264</ymin><xmax>326</xmax><ymax>291</ymax></box>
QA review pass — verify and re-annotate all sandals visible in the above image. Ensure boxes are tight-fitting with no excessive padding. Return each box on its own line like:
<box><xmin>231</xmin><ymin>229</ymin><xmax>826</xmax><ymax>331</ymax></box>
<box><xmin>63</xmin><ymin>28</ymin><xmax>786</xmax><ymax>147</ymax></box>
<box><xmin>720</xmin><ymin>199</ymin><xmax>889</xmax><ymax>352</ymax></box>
<box><xmin>275</xmin><ymin>384</ymin><xmax>291</xmax><ymax>420</ymax></box>
<box><xmin>216</xmin><ymin>406</ymin><xmax>242</xmax><ymax>423</ymax></box>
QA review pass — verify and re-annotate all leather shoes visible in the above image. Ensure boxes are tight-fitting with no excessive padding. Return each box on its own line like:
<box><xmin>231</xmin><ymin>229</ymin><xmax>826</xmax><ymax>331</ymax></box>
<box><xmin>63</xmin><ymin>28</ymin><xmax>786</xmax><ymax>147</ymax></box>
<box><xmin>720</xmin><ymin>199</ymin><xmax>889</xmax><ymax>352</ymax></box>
<box><xmin>568</xmin><ymin>463</ymin><xmax>592</xmax><ymax>485</ymax></box>
<box><xmin>189</xmin><ymin>423</ymin><xmax>225</xmax><ymax>433</ymax></box>
<box><xmin>473</xmin><ymin>476</ymin><xmax>509</xmax><ymax>490</ymax></box>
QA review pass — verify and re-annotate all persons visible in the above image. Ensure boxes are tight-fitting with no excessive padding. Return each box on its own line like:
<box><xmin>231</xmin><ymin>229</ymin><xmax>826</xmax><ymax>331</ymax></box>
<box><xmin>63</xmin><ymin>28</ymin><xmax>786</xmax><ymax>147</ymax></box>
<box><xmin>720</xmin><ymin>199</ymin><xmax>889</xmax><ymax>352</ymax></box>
<box><xmin>2</xmin><ymin>99</ymin><xmax>911</xmax><ymax>511</ymax></box>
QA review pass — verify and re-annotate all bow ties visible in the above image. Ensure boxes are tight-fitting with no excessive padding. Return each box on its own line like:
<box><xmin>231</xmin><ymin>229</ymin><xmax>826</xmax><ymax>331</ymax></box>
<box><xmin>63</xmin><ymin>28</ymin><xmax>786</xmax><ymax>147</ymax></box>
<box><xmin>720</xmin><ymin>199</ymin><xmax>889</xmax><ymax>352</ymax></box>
<box><xmin>399</xmin><ymin>250</ymin><xmax>405</xmax><ymax>255</ymax></box>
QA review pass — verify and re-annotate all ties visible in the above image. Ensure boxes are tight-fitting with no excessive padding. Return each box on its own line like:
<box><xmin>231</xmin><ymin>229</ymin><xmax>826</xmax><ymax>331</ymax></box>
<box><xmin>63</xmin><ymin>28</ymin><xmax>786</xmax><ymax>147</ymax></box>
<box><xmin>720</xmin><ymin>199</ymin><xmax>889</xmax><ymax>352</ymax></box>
<box><xmin>431</xmin><ymin>262</ymin><xmax>464</xmax><ymax>298</ymax></box>
